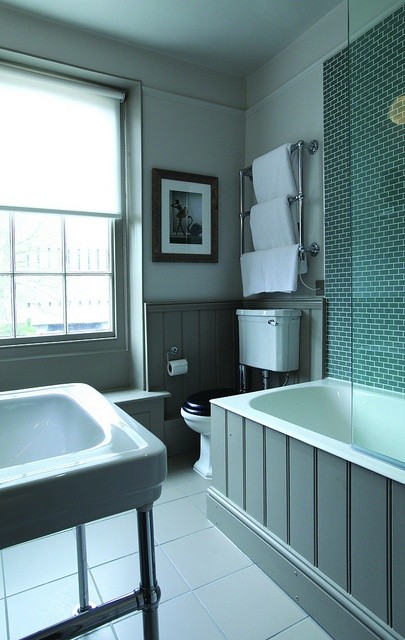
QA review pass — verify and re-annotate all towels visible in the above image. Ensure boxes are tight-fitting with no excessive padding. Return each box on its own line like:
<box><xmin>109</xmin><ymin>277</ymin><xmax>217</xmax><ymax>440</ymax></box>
<box><xmin>249</xmin><ymin>195</ymin><xmax>300</xmax><ymax>251</ymax></box>
<box><xmin>252</xmin><ymin>143</ymin><xmax>299</xmax><ymax>200</ymax></box>
<box><xmin>239</xmin><ymin>242</ymin><xmax>308</xmax><ymax>299</ymax></box>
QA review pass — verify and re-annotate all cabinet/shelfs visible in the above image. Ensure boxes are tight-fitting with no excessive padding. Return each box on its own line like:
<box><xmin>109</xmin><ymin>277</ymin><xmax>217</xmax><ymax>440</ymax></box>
<box><xmin>101</xmin><ymin>388</ymin><xmax>172</xmax><ymax>444</ymax></box>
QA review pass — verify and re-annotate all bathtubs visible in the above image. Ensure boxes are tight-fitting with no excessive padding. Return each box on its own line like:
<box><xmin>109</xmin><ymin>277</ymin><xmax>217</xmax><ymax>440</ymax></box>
<box><xmin>208</xmin><ymin>377</ymin><xmax>405</xmax><ymax>485</ymax></box>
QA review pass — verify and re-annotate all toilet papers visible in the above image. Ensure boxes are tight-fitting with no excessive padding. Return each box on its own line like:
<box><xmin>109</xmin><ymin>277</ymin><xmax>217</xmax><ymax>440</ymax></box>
<box><xmin>167</xmin><ymin>359</ymin><xmax>188</xmax><ymax>377</ymax></box>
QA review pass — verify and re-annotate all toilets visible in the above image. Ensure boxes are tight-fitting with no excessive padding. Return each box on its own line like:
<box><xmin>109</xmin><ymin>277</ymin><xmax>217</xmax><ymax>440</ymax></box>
<box><xmin>180</xmin><ymin>307</ymin><xmax>302</xmax><ymax>481</ymax></box>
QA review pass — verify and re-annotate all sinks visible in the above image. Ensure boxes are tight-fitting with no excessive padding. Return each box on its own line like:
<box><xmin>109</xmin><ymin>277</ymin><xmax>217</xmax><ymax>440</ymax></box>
<box><xmin>0</xmin><ymin>381</ymin><xmax>167</xmax><ymax>489</ymax></box>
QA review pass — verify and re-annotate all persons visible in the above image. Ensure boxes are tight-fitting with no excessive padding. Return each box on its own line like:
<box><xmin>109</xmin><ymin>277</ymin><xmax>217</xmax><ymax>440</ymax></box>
<box><xmin>170</xmin><ymin>199</ymin><xmax>187</xmax><ymax>236</ymax></box>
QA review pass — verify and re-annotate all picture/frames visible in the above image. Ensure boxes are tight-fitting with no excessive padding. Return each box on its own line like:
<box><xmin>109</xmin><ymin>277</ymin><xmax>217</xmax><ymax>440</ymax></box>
<box><xmin>151</xmin><ymin>168</ymin><xmax>218</xmax><ymax>262</ymax></box>
